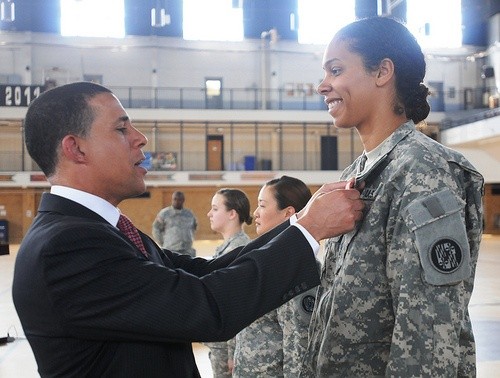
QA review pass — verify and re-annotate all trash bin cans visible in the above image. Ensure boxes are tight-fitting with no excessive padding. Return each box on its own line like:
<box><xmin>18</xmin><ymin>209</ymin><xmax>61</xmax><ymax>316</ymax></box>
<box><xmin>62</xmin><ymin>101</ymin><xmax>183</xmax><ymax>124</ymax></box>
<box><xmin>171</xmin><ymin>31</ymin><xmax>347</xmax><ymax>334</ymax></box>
<box><xmin>243</xmin><ymin>156</ymin><xmax>255</xmax><ymax>169</ymax></box>
<box><xmin>263</xmin><ymin>160</ymin><xmax>271</xmax><ymax>169</ymax></box>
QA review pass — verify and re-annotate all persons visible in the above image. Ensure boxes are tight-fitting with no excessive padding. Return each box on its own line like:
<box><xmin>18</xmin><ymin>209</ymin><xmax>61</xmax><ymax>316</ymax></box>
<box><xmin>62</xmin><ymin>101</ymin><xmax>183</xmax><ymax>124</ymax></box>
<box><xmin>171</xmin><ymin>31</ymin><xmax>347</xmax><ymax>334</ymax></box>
<box><xmin>152</xmin><ymin>191</ymin><xmax>197</xmax><ymax>258</ymax></box>
<box><xmin>299</xmin><ymin>17</ymin><xmax>484</xmax><ymax>378</ymax></box>
<box><xmin>199</xmin><ymin>188</ymin><xmax>253</xmax><ymax>378</ymax></box>
<box><xmin>232</xmin><ymin>175</ymin><xmax>322</xmax><ymax>378</ymax></box>
<box><xmin>12</xmin><ymin>81</ymin><xmax>364</xmax><ymax>378</ymax></box>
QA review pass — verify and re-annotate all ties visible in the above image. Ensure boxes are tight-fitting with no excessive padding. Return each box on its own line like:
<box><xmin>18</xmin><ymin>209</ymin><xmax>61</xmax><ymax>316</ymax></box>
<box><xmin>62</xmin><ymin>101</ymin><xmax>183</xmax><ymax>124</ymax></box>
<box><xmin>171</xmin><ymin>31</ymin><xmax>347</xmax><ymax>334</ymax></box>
<box><xmin>116</xmin><ymin>213</ymin><xmax>145</xmax><ymax>256</ymax></box>
